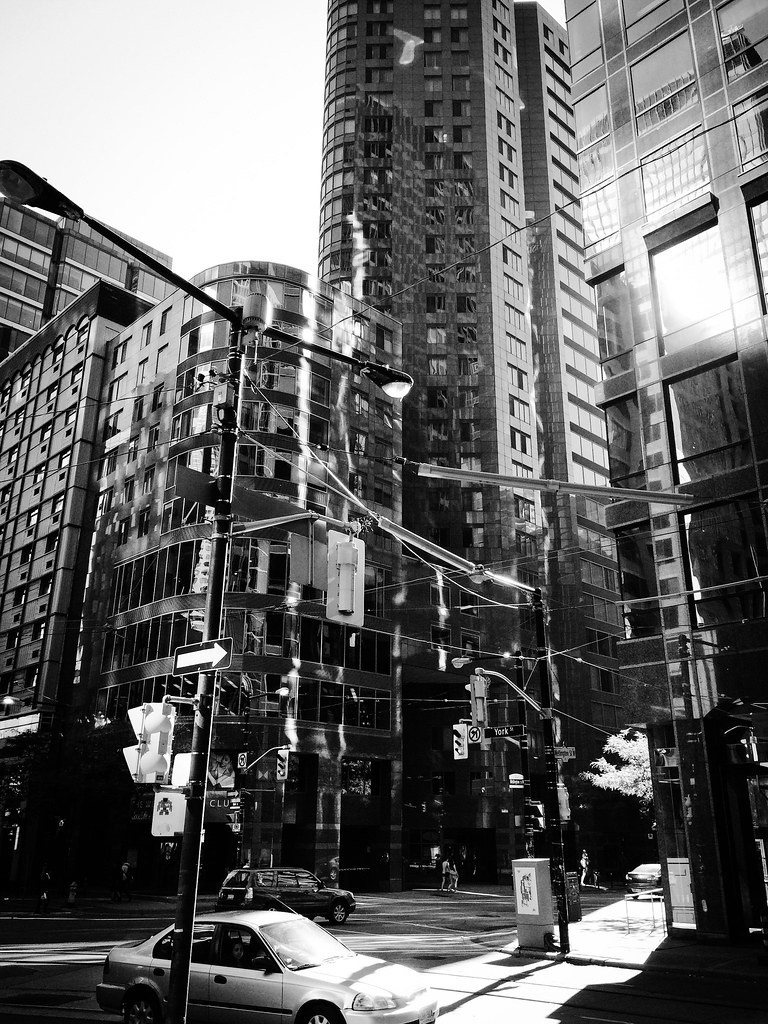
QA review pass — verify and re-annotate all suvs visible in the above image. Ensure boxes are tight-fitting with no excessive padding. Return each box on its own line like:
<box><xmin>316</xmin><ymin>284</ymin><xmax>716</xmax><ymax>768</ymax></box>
<box><xmin>218</xmin><ymin>869</ymin><xmax>356</xmax><ymax>923</ymax></box>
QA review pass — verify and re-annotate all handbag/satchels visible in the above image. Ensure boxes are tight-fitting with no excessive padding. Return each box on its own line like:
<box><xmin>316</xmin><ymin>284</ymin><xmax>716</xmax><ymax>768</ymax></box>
<box><xmin>449</xmin><ymin>869</ymin><xmax>457</xmax><ymax>876</ymax></box>
<box><xmin>446</xmin><ymin>868</ymin><xmax>450</xmax><ymax>872</ymax></box>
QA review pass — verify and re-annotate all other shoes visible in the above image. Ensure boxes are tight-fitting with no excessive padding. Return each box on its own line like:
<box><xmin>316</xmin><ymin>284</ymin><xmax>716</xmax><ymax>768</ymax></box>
<box><xmin>441</xmin><ymin>890</ymin><xmax>443</xmax><ymax>891</ymax></box>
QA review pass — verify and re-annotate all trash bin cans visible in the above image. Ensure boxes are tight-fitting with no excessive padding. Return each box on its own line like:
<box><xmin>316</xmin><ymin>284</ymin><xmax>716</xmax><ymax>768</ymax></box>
<box><xmin>555</xmin><ymin>871</ymin><xmax>582</xmax><ymax>923</ymax></box>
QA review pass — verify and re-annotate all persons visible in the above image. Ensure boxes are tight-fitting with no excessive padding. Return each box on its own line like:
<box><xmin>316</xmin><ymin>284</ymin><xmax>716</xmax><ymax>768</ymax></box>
<box><xmin>208</xmin><ymin>755</ymin><xmax>235</xmax><ymax>790</ymax></box>
<box><xmin>242</xmin><ymin>859</ymin><xmax>251</xmax><ymax>881</ymax></box>
<box><xmin>441</xmin><ymin>857</ymin><xmax>459</xmax><ymax>893</ymax></box>
<box><xmin>113</xmin><ymin>863</ymin><xmax>134</xmax><ymax>902</ymax></box>
<box><xmin>225</xmin><ymin>937</ymin><xmax>245</xmax><ymax>968</ymax></box>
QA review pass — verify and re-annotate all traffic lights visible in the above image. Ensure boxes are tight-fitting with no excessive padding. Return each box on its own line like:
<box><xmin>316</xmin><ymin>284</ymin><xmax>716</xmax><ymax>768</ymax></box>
<box><xmin>275</xmin><ymin>748</ymin><xmax>289</xmax><ymax>779</ymax></box>
<box><xmin>123</xmin><ymin>702</ymin><xmax>175</xmax><ymax>784</ymax></box>
<box><xmin>224</xmin><ymin>812</ymin><xmax>239</xmax><ymax>833</ymax></box>
<box><xmin>451</xmin><ymin>723</ymin><xmax>468</xmax><ymax>758</ymax></box>
<box><xmin>466</xmin><ymin>676</ymin><xmax>488</xmax><ymax>724</ymax></box>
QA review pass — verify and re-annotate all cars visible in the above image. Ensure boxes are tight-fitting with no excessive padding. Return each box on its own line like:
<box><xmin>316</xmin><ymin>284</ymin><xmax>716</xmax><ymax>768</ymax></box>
<box><xmin>625</xmin><ymin>862</ymin><xmax>662</xmax><ymax>900</ymax></box>
<box><xmin>315</xmin><ymin>855</ymin><xmax>339</xmax><ymax>880</ymax></box>
<box><xmin>96</xmin><ymin>909</ymin><xmax>441</xmax><ymax>1024</ymax></box>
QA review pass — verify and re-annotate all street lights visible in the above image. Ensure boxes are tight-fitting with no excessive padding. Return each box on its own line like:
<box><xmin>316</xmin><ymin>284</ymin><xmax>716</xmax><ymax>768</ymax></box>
<box><xmin>238</xmin><ymin>687</ymin><xmax>290</xmax><ymax>868</ymax></box>
<box><xmin>1</xmin><ymin>696</ymin><xmax>67</xmax><ymax>907</ymax></box>
<box><xmin>0</xmin><ymin>158</ymin><xmax>413</xmax><ymax>1023</ymax></box>
